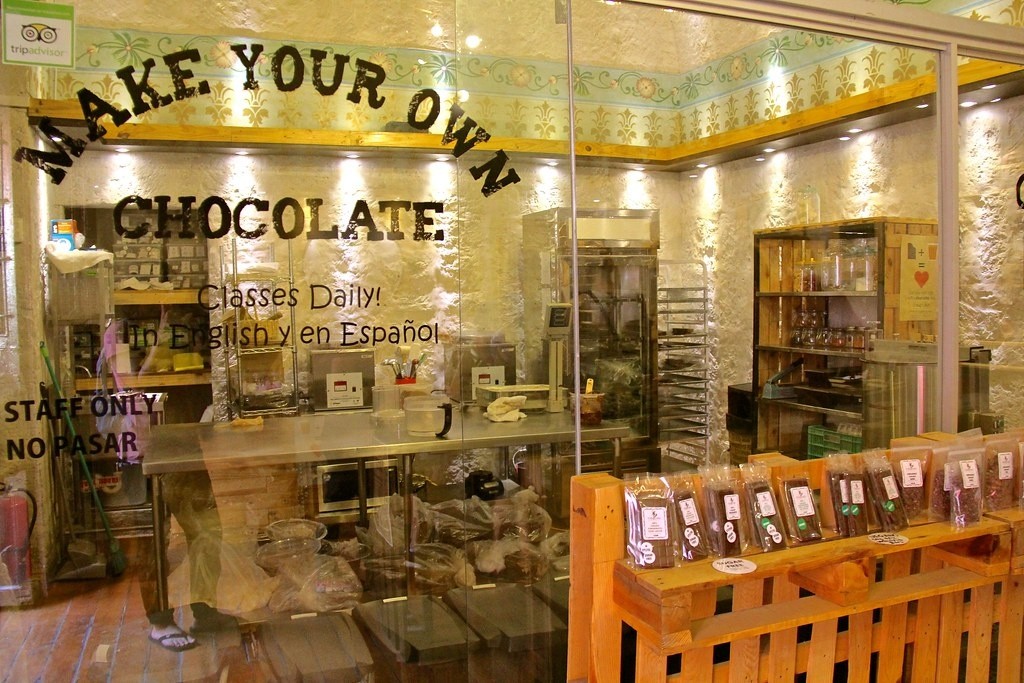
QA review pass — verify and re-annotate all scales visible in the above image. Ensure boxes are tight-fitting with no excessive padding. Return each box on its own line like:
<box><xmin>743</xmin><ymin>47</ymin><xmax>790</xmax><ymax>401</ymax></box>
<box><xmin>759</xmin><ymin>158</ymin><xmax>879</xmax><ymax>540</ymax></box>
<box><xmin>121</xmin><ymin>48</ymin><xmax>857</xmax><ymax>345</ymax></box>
<box><xmin>475</xmin><ymin>303</ymin><xmax>572</xmax><ymax>413</ymax></box>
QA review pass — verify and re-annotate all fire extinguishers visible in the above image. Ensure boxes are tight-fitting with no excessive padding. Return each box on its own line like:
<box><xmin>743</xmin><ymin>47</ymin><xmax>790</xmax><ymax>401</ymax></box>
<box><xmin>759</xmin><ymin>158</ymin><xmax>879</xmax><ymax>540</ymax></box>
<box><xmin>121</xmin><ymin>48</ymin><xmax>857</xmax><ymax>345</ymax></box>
<box><xmin>0</xmin><ymin>481</ymin><xmax>37</xmax><ymax>583</ymax></box>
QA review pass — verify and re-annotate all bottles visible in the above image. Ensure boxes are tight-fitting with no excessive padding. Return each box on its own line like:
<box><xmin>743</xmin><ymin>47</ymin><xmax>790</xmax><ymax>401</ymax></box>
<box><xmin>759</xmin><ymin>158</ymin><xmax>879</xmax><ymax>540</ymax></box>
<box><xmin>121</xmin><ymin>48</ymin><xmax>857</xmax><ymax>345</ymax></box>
<box><xmin>790</xmin><ymin>308</ymin><xmax>866</xmax><ymax>349</ymax></box>
<box><xmin>795</xmin><ymin>184</ymin><xmax>821</xmax><ymax>225</ymax></box>
<box><xmin>793</xmin><ymin>239</ymin><xmax>879</xmax><ymax>291</ymax></box>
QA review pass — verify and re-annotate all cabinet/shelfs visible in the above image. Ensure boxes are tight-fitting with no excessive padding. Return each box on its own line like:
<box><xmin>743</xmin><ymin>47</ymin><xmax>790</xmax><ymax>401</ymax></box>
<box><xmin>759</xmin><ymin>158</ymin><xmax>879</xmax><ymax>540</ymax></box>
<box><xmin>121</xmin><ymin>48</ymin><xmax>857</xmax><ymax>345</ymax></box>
<box><xmin>751</xmin><ymin>215</ymin><xmax>939</xmax><ymax>459</ymax></box>
<box><xmin>657</xmin><ymin>259</ymin><xmax>710</xmax><ymax>472</ymax></box>
<box><xmin>216</xmin><ymin>236</ymin><xmax>300</xmax><ymax>421</ymax></box>
<box><xmin>57</xmin><ymin>287</ymin><xmax>210</xmax><ymax>550</ymax></box>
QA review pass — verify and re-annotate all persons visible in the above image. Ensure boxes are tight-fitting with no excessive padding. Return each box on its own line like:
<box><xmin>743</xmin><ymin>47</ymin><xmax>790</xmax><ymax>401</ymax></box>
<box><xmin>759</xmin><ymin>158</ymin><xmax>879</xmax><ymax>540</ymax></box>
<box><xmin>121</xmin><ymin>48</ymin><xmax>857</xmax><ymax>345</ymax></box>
<box><xmin>105</xmin><ymin>259</ymin><xmax>248</xmax><ymax>652</ymax></box>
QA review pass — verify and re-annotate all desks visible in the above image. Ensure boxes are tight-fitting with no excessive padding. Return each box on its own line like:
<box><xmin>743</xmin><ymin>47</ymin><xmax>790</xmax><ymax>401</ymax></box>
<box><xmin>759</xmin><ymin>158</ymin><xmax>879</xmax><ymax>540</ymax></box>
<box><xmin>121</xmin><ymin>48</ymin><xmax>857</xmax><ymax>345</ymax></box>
<box><xmin>145</xmin><ymin>408</ymin><xmax>630</xmax><ymax>613</ymax></box>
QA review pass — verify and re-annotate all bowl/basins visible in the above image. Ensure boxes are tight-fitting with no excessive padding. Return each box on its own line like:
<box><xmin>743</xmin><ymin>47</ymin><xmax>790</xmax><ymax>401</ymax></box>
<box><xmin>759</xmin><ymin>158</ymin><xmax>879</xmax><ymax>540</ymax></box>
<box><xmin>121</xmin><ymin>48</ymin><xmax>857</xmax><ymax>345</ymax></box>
<box><xmin>255</xmin><ymin>518</ymin><xmax>371</xmax><ymax>592</ymax></box>
<box><xmin>371</xmin><ymin>409</ymin><xmax>406</xmax><ymax>432</ymax></box>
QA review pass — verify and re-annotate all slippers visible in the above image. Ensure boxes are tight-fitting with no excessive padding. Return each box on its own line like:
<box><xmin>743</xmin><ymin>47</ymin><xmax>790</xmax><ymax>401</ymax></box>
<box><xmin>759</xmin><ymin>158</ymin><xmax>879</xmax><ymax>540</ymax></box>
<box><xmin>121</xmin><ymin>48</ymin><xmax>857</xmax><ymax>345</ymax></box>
<box><xmin>148</xmin><ymin>631</ymin><xmax>198</xmax><ymax>652</ymax></box>
<box><xmin>189</xmin><ymin>615</ymin><xmax>248</xmax><ymax>633</ymax></box>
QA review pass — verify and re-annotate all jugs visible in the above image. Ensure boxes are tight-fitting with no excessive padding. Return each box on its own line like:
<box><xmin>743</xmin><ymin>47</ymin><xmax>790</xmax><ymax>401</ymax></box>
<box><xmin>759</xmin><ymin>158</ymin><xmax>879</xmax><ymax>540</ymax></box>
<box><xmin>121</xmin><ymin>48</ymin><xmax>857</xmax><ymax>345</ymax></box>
<box><xmin>403</xmin><ymin>394</ymin><xmax>452</xmax><ymax>437</ymax></box>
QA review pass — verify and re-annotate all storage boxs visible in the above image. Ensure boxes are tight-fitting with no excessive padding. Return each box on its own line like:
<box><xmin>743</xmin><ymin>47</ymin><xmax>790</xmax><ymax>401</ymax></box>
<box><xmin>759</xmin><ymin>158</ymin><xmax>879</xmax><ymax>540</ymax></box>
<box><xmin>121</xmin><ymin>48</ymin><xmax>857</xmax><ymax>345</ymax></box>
<box><xmin>806</xmin><ymin>424</ymin><xmax>861</xmax><ymax>457</ymax></box>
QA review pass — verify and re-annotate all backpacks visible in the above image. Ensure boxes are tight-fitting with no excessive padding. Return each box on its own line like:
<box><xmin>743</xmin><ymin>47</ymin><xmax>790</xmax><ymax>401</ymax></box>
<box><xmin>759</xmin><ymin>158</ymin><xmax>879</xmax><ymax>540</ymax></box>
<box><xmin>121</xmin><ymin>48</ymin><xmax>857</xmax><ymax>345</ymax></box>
<box><xmin>95</xmin><ymin>317</ymin><xmax>134</xmax><ymax>439</ymax></box>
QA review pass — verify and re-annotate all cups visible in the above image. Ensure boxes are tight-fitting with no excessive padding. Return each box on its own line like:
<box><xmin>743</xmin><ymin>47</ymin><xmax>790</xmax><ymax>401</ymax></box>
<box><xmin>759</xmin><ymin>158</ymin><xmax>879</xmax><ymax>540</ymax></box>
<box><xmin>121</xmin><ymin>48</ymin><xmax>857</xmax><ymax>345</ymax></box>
<box><xmin>569</xmin><ymin>391</ymin><xmax>604</xmax><ymax>425</ymax></box>
<box><xmin>372</xmin><ymin>385</ymin><xmax>401</xmax><ymax>412</ymax></box>
<box><xmin>396</xmin><ymin>378</ymin><xmax>416</xmax><ymax>385</ymax></box>
